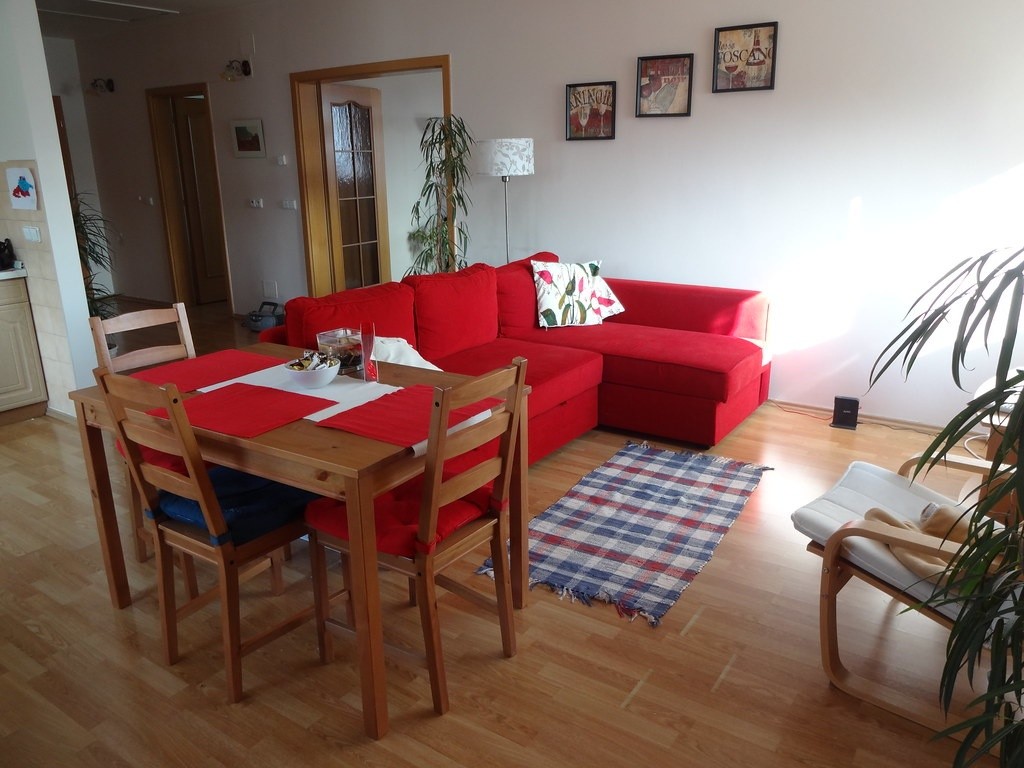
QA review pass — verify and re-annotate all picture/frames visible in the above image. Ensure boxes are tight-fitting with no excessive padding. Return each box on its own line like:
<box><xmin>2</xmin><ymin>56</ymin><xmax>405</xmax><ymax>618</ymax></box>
<box><xmin>565</xmin><ymin>80</ymin><xmax>617</xmax><ymax>141</ymax></box>
<box><xmin>711</xmin><ymin>21</ymin><xmax>778</xmax><ymax>93</ymax></box>
<box><xmin>229</xmin><ymin>119</ymin><xmax>267</xmax><ymax>158</ymax></box>
<box><xmin>635</xmin><ymin>53</ymin><xmax>694</xmax><ymax>118</ymax></box>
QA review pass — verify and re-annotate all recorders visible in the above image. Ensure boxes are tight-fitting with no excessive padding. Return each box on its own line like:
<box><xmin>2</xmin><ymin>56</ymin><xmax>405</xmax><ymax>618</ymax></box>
<box><xmin>247</xmin><ymin>301</ymin><xmax>284</xmax><ymax>332</ymax></box>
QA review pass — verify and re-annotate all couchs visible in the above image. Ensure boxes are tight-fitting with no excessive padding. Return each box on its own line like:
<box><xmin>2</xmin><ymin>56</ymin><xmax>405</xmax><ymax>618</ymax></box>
<box><xmin>258</xmin><ymin>251</ymin><xmax>773</xmax><ymax>513</ymax></box>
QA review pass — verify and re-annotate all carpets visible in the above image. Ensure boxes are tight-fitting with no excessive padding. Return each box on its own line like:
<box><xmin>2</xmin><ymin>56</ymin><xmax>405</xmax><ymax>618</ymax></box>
<box><xmin>474</xmin><ymin>438</ymin><xmax>775</xmax><ymax>628</ymax></box>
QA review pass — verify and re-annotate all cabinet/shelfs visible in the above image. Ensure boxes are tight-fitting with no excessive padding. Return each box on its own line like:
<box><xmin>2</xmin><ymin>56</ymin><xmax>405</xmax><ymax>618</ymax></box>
<box><xmin>0</xmin><ymin>270</ymin><xmax>49</xmax><ymax>426</ymax></box>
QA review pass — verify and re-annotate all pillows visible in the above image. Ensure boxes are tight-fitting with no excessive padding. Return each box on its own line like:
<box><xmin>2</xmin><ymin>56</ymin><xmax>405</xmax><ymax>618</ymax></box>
<box><xmin>359</xmin><ymin>334</ymin><xmax>446</xmax><ymax>373</ymax></box>
<box><xmin>530</xmin><ymin>259</ymin><xmax>625</xmax><ymax>328</ymax></box>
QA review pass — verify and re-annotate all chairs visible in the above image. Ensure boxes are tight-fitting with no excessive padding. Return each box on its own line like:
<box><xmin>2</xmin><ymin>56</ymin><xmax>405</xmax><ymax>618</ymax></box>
<box><xmin>89</xmin><ymin>365</ymin><xmax>355</xmax><ymax>703</ymax></box>
<box><xmin>88</xmin><ymin>302</ymin><xmax>201</xmax><ymax>607</ymax></box>
<box><xmin>806</xmin><ymin>451</ymin><xmax>1024</xmax><ymax>758</ymax></box>
<box><xmin>305</xmin><ymin>355</ymin><xmax>528</xmax><ymax>715</ymax></box>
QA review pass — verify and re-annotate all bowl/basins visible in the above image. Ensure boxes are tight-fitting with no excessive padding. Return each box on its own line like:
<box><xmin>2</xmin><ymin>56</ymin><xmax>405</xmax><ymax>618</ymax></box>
<box><xmin>285</xmin><ymin>356</ymin><xmax>341</xmax><ymax>388</ymax></box>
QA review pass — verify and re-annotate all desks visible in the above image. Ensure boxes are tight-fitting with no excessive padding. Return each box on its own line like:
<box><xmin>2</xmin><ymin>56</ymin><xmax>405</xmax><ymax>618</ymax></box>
<box><xmin>68</xmin><ymin>342</ymin><xmax>533</xmax><ymax>740</ymax></box>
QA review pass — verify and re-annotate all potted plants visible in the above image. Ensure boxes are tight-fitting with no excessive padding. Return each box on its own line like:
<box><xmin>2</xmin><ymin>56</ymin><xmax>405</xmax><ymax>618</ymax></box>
<box><xmin>69</xmin><ymin>191</ymin><xmax>122</xmax><ymax>358</ymax></box>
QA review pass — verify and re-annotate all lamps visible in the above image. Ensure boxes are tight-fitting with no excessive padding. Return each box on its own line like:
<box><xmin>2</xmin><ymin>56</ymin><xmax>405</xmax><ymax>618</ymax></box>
<box><xmin>475</xmin><ymin>137</ymin><xmax>536</xmax><ymax>263</ymax></box>
<box><xmin>219</xmin><ymin>59</ymin><xmax>252</xmax><ymax>83</ymax></box>
<box><xmin>85</xmin><ymin>78</ymin><xmax>114</xmax><ymax>96</ymax></box>
